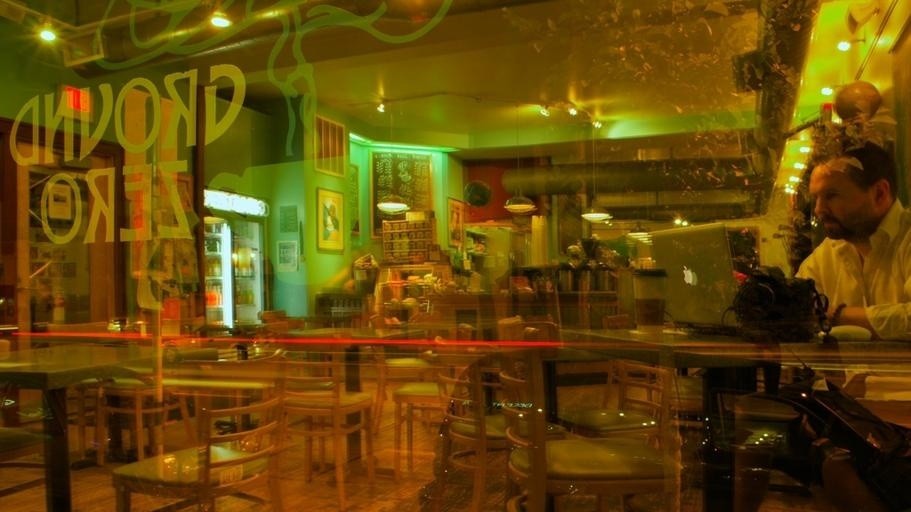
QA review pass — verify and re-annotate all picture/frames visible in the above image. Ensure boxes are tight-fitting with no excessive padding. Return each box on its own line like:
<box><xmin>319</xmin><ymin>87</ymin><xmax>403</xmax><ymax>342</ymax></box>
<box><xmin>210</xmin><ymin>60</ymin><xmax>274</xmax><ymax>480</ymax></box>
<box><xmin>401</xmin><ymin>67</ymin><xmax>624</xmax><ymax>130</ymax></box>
<box><xmin>315</xmin><ymin>187</ymin><xmax>344</xmax><ymax>252</ymax></box>
<box><xmin>447</xmin><ymin>195</ymin><xmax>466</xmax><ymax>248</ymax></box>
<box><xmin>369</xmin><ymin>150</ymin><xmax>435</xmax><ymax>241</ymax></box>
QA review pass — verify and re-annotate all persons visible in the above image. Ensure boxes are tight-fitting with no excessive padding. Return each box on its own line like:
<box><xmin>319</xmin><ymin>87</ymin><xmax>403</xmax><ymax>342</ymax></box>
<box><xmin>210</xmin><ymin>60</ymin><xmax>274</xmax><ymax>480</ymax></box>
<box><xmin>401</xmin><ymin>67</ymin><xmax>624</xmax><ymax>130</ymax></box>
<box><xmin>794</xmin><ymin>141</ymin><xmax>911</xmax><ymax>347</ymax></box>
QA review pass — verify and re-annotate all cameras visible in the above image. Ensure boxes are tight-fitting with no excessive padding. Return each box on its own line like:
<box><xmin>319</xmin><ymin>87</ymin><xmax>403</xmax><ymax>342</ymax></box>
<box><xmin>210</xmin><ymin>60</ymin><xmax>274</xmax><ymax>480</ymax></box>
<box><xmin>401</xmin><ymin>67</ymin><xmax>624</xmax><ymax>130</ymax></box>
<box><xmin>732</xmin><ymin>264</ymin><xmax>817</xmax><ymax>339</ymax></box>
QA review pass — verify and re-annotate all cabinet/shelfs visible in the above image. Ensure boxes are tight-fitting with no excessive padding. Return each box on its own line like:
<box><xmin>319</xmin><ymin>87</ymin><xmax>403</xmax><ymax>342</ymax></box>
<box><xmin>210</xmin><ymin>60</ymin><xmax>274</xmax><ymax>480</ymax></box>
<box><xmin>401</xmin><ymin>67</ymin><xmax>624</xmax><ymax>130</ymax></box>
<box><xmin>466</xmin><ymin>231</ymin><xmax>487</xmax><ymax>257</ymax></box>
<box><xmin>382</xmin><ymin>220</ymin><xmax>436</xmax><ymax>263</ymax></box>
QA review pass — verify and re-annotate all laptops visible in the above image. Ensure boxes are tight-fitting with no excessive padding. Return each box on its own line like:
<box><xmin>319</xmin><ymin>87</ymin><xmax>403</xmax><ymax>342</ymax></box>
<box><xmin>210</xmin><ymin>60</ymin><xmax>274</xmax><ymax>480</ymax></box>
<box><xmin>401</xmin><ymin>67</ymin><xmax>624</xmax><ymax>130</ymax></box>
<box><xmin>652</xmin><ymin>224</ymin><xmax>743</xmax><ymax>329</ymax></box>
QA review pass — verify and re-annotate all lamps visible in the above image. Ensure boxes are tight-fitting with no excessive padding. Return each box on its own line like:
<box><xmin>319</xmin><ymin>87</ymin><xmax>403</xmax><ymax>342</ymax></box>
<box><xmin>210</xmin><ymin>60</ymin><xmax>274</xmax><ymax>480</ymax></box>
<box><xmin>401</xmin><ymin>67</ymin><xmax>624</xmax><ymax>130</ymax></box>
<box><xmin>580</xmin><ymin>117</ymin><xmax>611</xmax><ymax>222</ymax></box>
<box><xmin>502</xmin><ymin>88</ymin><xmax>536</xmax><ymax>214</ymax></box>
<box><xmin>375</xmin><ymin>113</ymin><xmax>411</xmax><ymax>214</ymax></box>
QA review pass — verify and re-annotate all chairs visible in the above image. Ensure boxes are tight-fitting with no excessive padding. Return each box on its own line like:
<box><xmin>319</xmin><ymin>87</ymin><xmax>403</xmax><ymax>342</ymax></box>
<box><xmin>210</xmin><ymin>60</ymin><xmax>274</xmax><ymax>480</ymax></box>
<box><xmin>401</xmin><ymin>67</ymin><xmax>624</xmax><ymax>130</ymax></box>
<box><xmin>2</xmin><ymin>315</ymin><xmax>910</xmax><ymax>428</ymax></box>
<box><xmin>500</xmin><ymin>367</ymin><xmax>678</xmax><ymax>510</ymax></box>
<box><xmin>426</xmin><ymin>350</ymin><xmax>566</xmax><ymax>509</ymax></box>
<box><xmin>107</xmin><ymin>396</ymin><xmax>285</xmax><ymax>510</ymax></box>
<box><xmin>95</xmin><ymin>332</ymin><xmax>191</xmax><ymax>470</ymax></box>
<box><xmin>271</xmin><ymin>354</ymin><xmax>373</xmax><ymax>507</ymax></box>
<box><xmin>391</xmin><ymin>325</ymin><xmax>476</xmax><ymax>478</ymax></box>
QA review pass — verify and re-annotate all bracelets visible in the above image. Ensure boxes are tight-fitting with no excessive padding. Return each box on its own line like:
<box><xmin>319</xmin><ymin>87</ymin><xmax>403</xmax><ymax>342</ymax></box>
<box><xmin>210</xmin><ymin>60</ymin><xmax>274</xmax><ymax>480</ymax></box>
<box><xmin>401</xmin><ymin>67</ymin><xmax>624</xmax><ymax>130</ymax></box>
<box><xmin>830</xmin><ymin>303</ymin><xmax>847</xmax><ymax>325</ymax></box>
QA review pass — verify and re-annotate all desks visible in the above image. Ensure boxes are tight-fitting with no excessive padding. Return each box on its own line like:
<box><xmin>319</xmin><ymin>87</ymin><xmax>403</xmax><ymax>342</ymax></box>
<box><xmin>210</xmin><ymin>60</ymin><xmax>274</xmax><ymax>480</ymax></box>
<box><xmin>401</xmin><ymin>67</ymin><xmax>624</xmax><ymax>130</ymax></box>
<box><xmin>5</xmin><ymin>344</ymin><xmax>221</xmax><ymax>508</ymax></box>
<box><xmin>592</xmin><ymin>335</ymin><xmax>909</xmax><ymax>512</ymax></box>
